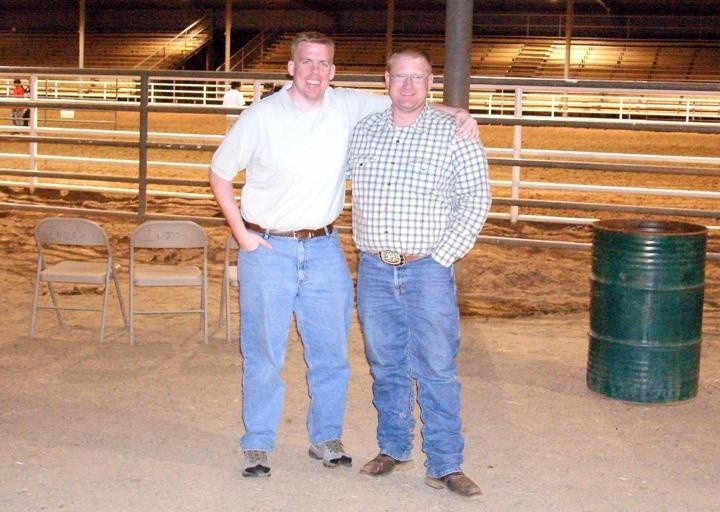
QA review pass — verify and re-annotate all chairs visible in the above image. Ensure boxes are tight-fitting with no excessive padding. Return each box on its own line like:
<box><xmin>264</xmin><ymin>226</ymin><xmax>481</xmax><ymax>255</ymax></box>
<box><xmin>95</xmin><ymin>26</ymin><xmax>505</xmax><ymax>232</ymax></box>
<box><xmin>30</xmin><ymin>217</ymin><xmax>127</xmax><ymax>343</ymax></box>
<box><xmin>219</xmin><ymin>230</ymin><xmax>241</xmax><ymax>343</ymax></box>
<box><xmin>128</xmin><ymin>221</ymin><xmax>208</xmax><ymax>345</ymax></box>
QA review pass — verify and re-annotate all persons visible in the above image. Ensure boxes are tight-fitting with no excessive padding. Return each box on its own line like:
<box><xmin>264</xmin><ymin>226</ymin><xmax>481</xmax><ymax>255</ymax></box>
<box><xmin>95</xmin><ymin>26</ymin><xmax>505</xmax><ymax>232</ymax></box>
<box><xmin>210</xmin><ymin>30</ymin><xmax>479</xmax><ymax>478</ymax></box>
<box><xmin>11</xmin><ymin>79</ymin><xmax>24</xmax><ymax>124</ymax></box>
<box><xmin>222</xmin><ymin>81</ymin><xmax>245</xmax><ymax>116</ymax></box>
<box><xmin>346</xmin><ymin>46</ymin><xmax>493</xmax><ymax>500</ymax></box>
<box><xmin>21</xmin><ymin>82</ymin><xmax>30</xmax><ymax>125</ymax></box>
<box><xmin>261</xmin><ymin>83</ymin><xmax>275</xmax><ymax>98</ymax></box>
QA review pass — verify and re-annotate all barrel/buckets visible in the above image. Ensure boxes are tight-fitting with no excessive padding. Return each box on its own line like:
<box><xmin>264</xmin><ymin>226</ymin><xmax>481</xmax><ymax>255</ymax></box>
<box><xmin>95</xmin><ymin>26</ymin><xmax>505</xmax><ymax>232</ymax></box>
<box><xmin>586</xmin><ymin>219</ymin><xmax>707</xmax><ymax>404</ymax></box>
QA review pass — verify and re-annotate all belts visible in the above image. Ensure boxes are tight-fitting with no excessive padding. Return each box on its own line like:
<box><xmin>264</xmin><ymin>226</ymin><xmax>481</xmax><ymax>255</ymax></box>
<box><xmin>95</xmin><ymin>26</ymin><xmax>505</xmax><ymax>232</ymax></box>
<box><xmin>371</xmin><ymin>250</ymin><xmax>428</xmax><ymax>266</ymax></box>
<box><xmin>244</xmin><ymin>222</ymin><xmax>333</xmax><ymax>237</ymax></box>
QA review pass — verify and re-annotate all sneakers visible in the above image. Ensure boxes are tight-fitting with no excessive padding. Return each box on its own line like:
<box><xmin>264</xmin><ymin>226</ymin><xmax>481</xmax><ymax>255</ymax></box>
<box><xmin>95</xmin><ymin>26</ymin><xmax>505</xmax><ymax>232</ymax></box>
<box><xmin>426</xmin><ymin>471</ymin><xmax>482</xmax><ymax>497</ymax></box>
<box><xmin>308</xmin><ymin>437</ymin><xmax>353</xmax><ymax>468</ymax></box>
<box><xmin>361</xmin><ymin>454</ymin><xmax>398</xmax><ymax>474</ymax></box>
<box><xmin>242</xmin><ymin>450</ymin><xmax>272</xmax><ymax>478</ymax></box>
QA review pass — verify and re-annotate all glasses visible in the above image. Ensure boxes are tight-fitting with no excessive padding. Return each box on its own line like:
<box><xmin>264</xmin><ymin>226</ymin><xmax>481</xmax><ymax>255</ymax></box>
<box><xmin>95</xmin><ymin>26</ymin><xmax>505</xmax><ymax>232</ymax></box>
<box><xmin>388</xmin><ymin>71</ymin><xmax>431</xmax><ymax>83</ymax></box>
